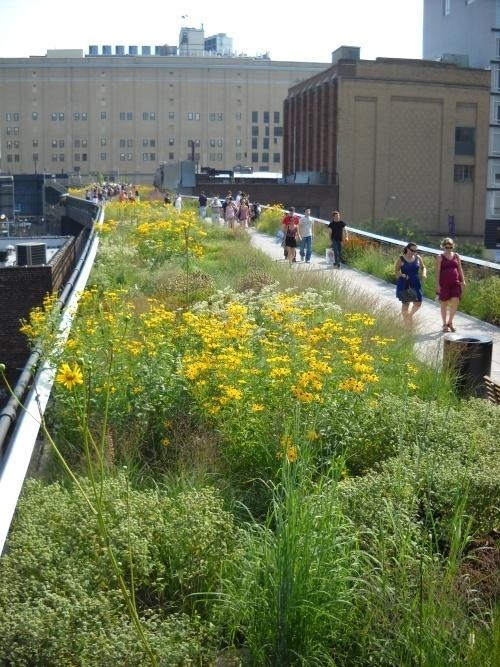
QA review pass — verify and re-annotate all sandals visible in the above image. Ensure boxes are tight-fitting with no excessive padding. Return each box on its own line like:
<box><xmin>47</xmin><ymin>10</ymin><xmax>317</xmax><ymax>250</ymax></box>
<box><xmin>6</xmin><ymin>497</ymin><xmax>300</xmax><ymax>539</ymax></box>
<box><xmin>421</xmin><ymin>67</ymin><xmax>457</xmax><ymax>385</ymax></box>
<box><xmin>442</xmin><ymin>322</ymin><xmax>456</xmax><ymax>333</ymax></box>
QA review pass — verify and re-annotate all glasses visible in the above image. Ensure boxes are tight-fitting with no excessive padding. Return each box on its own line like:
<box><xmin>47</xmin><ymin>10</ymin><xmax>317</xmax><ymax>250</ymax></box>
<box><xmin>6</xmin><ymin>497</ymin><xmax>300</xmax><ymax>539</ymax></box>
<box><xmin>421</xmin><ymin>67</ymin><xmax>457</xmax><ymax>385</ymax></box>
<box><xmin>407</xmin><ymin>247</ymin><xmax>417</xmax><ymax>253</ymax></box>
<box><xmin>444</xmin><ymin>247</ymin><xmax>453</xmax><ymax>250</ymax></box>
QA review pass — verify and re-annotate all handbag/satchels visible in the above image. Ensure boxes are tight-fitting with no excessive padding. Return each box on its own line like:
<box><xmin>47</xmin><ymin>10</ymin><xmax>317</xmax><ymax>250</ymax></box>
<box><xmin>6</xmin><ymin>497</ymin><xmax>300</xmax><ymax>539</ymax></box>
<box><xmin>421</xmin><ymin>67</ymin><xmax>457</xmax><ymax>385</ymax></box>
<box><xmin>400</xmin><ymin>287</ymin><xmax>418</xmax><ymax>303</ymax></box>
<box><xmin>281</xmin><ymin>240</ymin><xmax>286</xmax><ymax>247</ymax></box>
<box><xmin>326</xmin><ymin>247</ymin><xmax>336</xmax><ymax>264</ymax></box>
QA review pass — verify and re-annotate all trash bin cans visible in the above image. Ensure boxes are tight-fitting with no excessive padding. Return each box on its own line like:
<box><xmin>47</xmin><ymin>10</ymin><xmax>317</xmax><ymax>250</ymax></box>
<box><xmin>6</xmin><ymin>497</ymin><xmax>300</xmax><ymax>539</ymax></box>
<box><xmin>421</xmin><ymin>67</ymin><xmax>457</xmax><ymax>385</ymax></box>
<box><xmin>443</xmin><ymin>335</ymin><xmax>493</xmax><ymax>398</ymax></box>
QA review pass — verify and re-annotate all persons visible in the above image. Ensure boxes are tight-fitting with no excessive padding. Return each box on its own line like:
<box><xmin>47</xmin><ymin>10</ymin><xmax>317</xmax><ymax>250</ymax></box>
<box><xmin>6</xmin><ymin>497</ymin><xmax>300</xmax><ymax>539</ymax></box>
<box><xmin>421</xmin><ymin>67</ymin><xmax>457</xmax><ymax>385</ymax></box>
<box><xmin>281</xmin><ymin>206</ymin><xmax>303</xmax><ymax>263</ymax></box>
<box><xmin>198</xmin><ymin>189</ymin><xmax>262</xmax><ymax>233</ymax></box>
<box><xmin>174</xmin><ymin>191</ymin><xmax>184</xmax><ymax>211</ymax></box>
<box><xmin>434</xmin><ymin>236</ymin><xmax>467</xmax><ymax>333</ymax></box>
<box><xmin>283</xmin><ymin>216</ymin><xmax>303</xmax><ymax>265</ymax></box>
<box><xmin>84</xmin><ymin>184</ymin><xmax>140</xmax><ymax>204</ymax></box>
<box><xmin>327</xmin><ymin>211</ymin><xmax>349</xmax><ymax>267</ymax></box>
<box><xmin>297</xmin><ymin>208</ymin><xmax>314</xmax><ymax>263</ymax></box>
<box><xmin>394</xmin><ymin>241</ymin><xmax>427</xmax><ymax>327</ymax></box>
